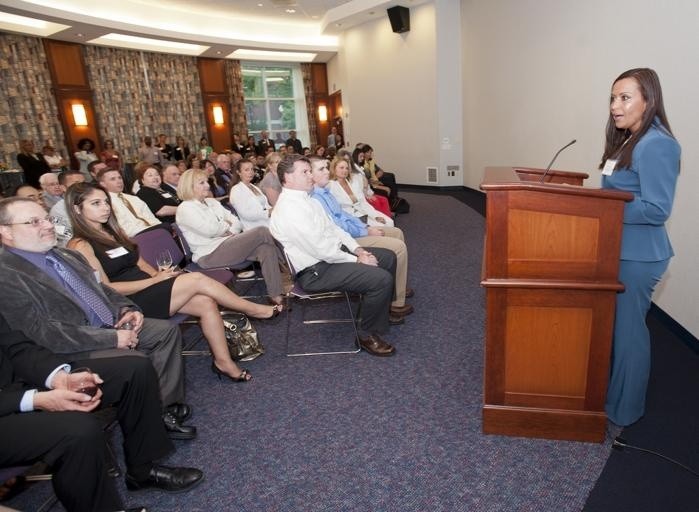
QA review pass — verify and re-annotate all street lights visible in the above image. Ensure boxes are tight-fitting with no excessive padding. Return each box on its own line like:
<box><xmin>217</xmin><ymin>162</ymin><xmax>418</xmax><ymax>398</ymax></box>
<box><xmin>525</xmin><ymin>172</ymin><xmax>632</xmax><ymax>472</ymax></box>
<box><xmin>220</xmin><ymin>311</ymin><xmax>264</xmax><ymax>362</ymax></box>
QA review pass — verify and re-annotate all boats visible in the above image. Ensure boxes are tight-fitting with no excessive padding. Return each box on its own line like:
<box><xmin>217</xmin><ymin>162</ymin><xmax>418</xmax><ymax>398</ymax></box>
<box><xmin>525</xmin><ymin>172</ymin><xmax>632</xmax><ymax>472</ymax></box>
<box><xmin>386</xmin><ymin>5</ymin><xmax>411</xmax><ymax>34</ymax></box>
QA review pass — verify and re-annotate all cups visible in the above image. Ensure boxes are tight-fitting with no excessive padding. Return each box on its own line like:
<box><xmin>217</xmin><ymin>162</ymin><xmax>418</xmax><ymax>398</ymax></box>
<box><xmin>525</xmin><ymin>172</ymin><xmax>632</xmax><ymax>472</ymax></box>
<box><xmin>3</xmin><ymin>215</ymin><xmax>55</xmax><ymax>226</ymax></box>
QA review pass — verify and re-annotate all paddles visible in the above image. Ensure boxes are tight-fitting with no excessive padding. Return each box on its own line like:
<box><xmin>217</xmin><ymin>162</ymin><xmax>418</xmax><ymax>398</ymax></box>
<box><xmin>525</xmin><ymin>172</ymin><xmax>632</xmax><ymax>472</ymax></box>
<box><xmin>0</xmin><ymin>191</ymin><xmax>623</xmax><ymax>512</ymax></box>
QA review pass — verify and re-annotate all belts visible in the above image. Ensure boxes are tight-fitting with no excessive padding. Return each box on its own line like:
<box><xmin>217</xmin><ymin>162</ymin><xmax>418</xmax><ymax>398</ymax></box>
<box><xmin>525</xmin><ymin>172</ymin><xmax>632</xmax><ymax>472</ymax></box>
<box><xmin>69</xmin><ymin>249</ymin><xmax>172</xmax><ymax>402</ymax></box>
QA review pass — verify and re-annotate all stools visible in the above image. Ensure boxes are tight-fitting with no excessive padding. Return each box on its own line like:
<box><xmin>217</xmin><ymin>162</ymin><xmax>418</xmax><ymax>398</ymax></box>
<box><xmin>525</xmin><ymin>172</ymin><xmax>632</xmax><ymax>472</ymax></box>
<box><xmin>295</xmin><ymin>261</ymin><xmax>322</xmax><ymax>278</ymax></box>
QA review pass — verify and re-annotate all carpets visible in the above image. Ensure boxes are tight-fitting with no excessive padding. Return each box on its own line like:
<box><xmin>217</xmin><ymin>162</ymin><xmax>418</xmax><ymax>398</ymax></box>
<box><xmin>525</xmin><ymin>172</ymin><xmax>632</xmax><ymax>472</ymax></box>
<box><xmin>46</xmin><ymin>255</ymin><xmax>113</xmax><ymax>327</ymax></box>
<box><xmin>119</xmin><ymin>194</ymin><xmax>150</xmax><ymax>226</ymax></box>
<box><xmin>0</xmin><ymin>191</ymin><xmax>623</xmax><ymax>512</ymax></box>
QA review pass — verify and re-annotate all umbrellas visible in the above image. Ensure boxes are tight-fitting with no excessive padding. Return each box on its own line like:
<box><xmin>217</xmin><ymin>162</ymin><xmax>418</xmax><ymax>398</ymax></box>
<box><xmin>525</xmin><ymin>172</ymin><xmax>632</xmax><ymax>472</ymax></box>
<box><xmin>355</xmin><ymin>335</ymin><xmax>396</xmax><ymax>357</ymax></box>
<box><xmin>390</xmin><ymin>289</ymin><xmax>414</xmax><ymax>325</ymax></box>
<box><xmin>124</xmin><ymin>463</ymin><xmax>203</xmax><ymax>492</ymax></box>
<box><xmin>163</xmin><ymin>403</ymin><xmax>196</xmax><ymax>440</ymax></box>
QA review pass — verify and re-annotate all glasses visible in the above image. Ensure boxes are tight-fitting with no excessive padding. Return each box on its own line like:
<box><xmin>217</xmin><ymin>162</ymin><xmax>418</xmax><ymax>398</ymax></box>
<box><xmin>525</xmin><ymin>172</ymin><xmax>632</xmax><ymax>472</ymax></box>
<box><xmin>71</xmin><ymin>104</ymin><xmax>88</xmax><ymax>126</ymax></box>
<box><xmin>319</xmin><ymin>105</ymin><xmax>327</xmax><ymax>121</ymax></box>
<box><xmin>212</xmin><ymin>106</ymin><xmax>224</xmax><ymax>124</ymax></box>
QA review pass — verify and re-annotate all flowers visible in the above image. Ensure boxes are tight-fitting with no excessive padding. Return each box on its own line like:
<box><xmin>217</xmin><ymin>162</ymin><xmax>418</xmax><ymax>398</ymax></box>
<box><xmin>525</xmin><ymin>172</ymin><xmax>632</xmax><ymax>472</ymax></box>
<box><xmin>538</xmin><ymin>137</ymin><xmax>579</xmax><ymax>183</ymax></box>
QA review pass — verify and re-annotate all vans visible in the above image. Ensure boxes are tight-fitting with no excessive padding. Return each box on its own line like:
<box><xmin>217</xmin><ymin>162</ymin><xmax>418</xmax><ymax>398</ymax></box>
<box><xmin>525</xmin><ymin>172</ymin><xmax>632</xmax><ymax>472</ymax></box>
<box><xmin>211</xmin><ymin>361</ymin><xmax>252</xmax><ymax>382</ymax></box>
<box><xmin>247</xmin><ymin>304</ymin><xmax>282</xmax><ymax>321</ymax></box>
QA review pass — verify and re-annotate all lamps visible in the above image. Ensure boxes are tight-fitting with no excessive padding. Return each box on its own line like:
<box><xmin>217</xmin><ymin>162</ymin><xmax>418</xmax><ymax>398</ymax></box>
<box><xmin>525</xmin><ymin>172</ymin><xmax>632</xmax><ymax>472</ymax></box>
<box><xmin>71</xmin><ymin>104</ymin><xmax>88</xmax><ymax>126</ymax></box>
<box><xmin>319</xmin><ymin>105</ymin><xmax>327</xmax><ymax>121</ymax></box>
<box><xmin>212</xmin><ymin>106</ymin><xmax>224</xmax><ymax>124</ymax></box>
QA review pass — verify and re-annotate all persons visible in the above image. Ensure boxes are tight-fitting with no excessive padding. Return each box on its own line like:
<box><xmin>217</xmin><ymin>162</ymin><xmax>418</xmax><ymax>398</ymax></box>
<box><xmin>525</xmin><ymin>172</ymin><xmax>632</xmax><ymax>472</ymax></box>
<box><xmin>0</xmin><ymin>313</ymin><xmax>146</xmax><ymax>512</ymax></box>
<box><xmin>598</xmin><ymin>68</ymin><xmax>682</xmax><ymax>428</ymax></box>
<box><xmin>0</xmin><ymin>196</ymin><xmax>197</xmax><ymax>441</ymax></box>
<box><xmin>175</xmin><ymin>169</ymin><xmax>298</xmax><ymax>312</ymax></box>
<box><xmin>305</xmin><ymin>155</ymin><xmax>415</xmax><ymax>316</ymax></box>
<box><xmin>2</xmin><ymin>123</ymin><xmax>414</xmax><ymax>262</ymax></box>
<box><xmin>66</xmin><ymin>181</ymin><xmax>284</xmax><ymax>382</ymax></box>
<box><xmin>269</xmin><ymin>153</ymin><xmax>406</xmax><ymax>357</ymax></box>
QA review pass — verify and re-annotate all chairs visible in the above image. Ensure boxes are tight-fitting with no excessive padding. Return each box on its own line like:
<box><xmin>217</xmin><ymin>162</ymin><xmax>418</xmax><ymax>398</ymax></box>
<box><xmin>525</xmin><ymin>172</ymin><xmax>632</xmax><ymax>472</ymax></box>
<box><xmin>0</xmin><ymin>223</ymin><xmax>363</xmax><ymax>512</ymax></box>
